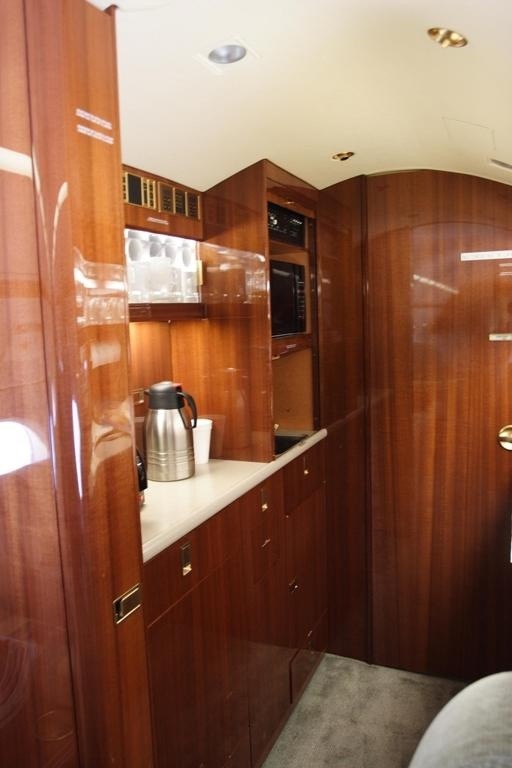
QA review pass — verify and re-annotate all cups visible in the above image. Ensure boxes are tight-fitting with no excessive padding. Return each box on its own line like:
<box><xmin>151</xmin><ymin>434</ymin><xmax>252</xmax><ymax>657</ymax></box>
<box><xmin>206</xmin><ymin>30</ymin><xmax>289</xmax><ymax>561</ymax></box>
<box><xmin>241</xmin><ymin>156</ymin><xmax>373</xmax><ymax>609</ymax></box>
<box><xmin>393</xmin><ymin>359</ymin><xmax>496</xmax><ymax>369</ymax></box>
<box><xmin>188</xmin><ymin>417</ymin><xmax>215</xmax><ymax>465</ymax></box>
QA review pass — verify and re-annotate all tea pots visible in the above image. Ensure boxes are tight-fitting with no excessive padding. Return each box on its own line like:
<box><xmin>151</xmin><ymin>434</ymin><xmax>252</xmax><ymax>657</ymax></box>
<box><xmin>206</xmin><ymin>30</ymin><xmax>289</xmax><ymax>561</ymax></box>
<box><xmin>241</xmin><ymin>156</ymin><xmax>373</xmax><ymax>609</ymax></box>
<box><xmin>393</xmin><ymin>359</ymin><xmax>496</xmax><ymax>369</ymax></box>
<box><xmin>141</xmin><ymin>380</ymin><xmax>200</xmax><ymax>485</ymax></box>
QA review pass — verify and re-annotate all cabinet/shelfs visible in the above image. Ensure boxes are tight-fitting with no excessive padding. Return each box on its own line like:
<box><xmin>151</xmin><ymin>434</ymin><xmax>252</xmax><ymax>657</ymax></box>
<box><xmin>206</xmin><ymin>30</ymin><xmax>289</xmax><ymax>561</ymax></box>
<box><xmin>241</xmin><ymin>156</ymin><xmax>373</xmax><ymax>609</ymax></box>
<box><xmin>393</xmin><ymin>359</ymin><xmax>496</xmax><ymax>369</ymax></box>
<box><xmin>171</xmin><ymin>159</ymin><xmax>321</xmax><ymax>463</ymax></box>
<box><xmin>0</xmin><ymin>0</ymin><xmax>156</xmax><ymax>767</ymax></box>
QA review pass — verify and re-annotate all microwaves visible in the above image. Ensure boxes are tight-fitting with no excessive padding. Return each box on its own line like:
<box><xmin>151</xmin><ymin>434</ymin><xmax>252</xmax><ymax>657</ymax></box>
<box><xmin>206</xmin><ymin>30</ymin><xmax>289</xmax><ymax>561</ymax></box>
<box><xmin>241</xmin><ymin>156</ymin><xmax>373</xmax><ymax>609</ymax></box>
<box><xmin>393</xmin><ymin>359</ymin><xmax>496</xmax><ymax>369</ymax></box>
<box><xmin>269</xmin><ymin>256</ymin><xmax>308</xmax><ymax>337</ymax></box>
